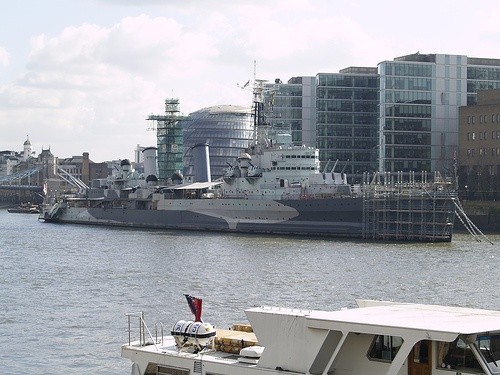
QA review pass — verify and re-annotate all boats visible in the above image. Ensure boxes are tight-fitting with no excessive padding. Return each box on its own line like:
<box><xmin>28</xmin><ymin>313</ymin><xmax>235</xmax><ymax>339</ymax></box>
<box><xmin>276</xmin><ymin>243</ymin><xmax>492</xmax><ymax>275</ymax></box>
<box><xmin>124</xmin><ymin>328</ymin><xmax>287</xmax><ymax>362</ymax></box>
<box><xmin>8</xmin><ymin>203</ymin><xmax>39</xmax><ymax>213</ymax></box>
<box><xmin>120</xmin><ymin>296</ymin><xmax>500</xmax><ymax>375</ymax></box>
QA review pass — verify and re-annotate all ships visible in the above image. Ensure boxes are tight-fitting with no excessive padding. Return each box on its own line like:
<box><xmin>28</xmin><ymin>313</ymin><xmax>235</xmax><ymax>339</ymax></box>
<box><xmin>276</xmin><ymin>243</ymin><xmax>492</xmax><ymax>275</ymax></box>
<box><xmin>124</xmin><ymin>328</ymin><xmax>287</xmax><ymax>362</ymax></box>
<box><xmin>32</xmin><ymin>80</ymin><xmax>457</xmax><ymax>241</ymax></box>
